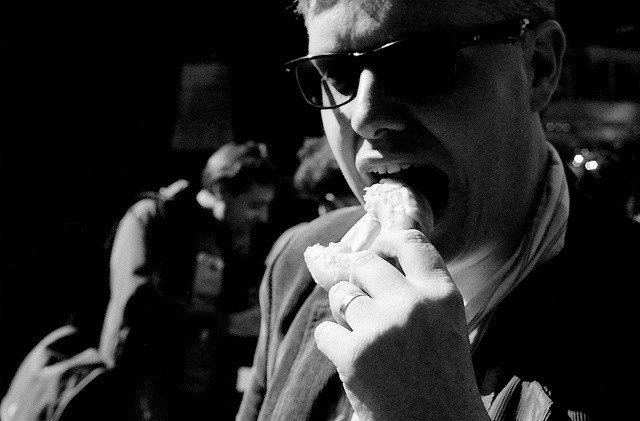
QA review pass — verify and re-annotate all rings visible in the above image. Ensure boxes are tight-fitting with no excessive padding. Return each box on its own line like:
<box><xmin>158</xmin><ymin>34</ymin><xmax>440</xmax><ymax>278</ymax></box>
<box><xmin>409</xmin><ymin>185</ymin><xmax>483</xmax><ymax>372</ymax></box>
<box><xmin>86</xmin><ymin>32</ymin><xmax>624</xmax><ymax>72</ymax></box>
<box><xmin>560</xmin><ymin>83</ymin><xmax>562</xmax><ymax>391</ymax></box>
<box><xmin>339</xmin><ymin>291</ymin><xmax>367</xmax><ymax>319</ymax></box>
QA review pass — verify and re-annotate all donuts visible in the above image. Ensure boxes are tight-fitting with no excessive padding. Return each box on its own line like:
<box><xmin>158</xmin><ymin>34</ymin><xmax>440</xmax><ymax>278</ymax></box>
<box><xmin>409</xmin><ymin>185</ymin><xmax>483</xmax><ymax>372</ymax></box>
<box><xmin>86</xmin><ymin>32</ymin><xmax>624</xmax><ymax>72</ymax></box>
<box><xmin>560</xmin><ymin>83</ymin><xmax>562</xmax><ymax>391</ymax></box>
<box><xmin>304</xmin><ymin>178</ymin><xmax>435</xmax><ymax>294</ymax></box>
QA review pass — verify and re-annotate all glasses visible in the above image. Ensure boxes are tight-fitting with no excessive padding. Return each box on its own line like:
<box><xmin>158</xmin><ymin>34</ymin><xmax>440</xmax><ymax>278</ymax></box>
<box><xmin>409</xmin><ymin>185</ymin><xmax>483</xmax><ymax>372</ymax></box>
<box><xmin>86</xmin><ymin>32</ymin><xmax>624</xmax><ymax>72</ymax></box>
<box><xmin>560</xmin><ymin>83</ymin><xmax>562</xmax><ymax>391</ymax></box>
<box><xmin>285</xmin><ymin>16</ymin><xmax>536</xmax><ymax>109</ymax></box>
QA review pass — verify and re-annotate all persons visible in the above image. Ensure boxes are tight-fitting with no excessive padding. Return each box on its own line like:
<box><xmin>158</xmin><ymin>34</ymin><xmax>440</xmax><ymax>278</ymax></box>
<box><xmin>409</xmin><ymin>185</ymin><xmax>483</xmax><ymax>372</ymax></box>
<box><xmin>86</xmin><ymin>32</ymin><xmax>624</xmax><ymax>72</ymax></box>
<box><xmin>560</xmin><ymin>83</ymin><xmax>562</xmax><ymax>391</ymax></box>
<box><xmin>230</xmin><ymin>1</ymin><xmax>639</xmax><ymax>420</ymax></box>
<box><xmin>293</xmin><ymin>131</ymin><xmax>362</xmax><ymax>216</ymax></box>
<box><xmin>97</xmin><ymin>139</ymin><xmax>278</xmax><ymax>416</ymax></box>
